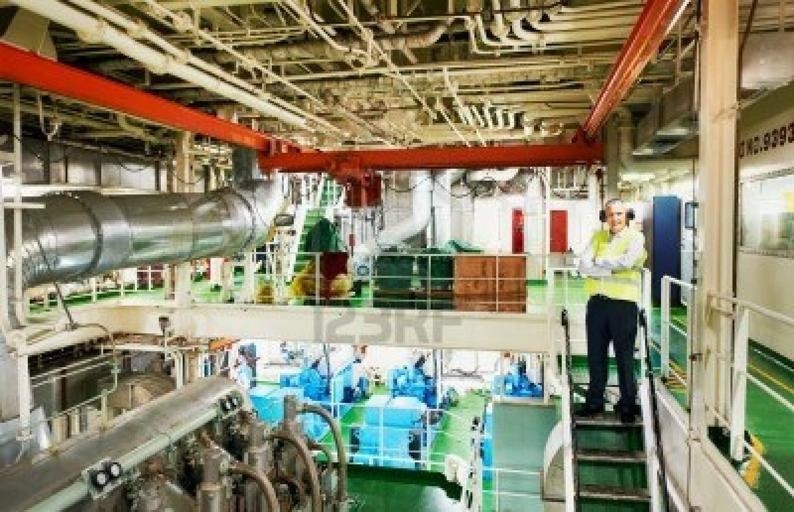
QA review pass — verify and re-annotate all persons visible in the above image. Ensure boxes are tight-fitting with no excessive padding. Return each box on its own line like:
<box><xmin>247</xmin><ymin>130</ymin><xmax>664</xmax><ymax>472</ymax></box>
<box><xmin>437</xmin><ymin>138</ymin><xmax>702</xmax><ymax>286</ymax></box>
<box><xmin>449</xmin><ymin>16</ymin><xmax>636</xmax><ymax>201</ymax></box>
<box><xmin>577</xmin><ymin>200</ymin><xmax>647</xmax><ymax>423</ymax></box>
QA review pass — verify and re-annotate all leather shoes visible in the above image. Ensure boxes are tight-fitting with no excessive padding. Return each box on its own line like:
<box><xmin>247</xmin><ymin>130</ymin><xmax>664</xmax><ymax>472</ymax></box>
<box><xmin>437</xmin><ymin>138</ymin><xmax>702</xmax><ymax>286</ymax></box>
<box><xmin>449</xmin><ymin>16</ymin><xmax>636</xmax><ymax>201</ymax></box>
<box><xmin>620</xmin><ymin>408</ymin><xmax>635</xmax><ymax>423</ymax></box>
<box><xmin>577</xmin><ymin>405</ymin><xmax>606</xmax><ymax>416</ymax></box>
